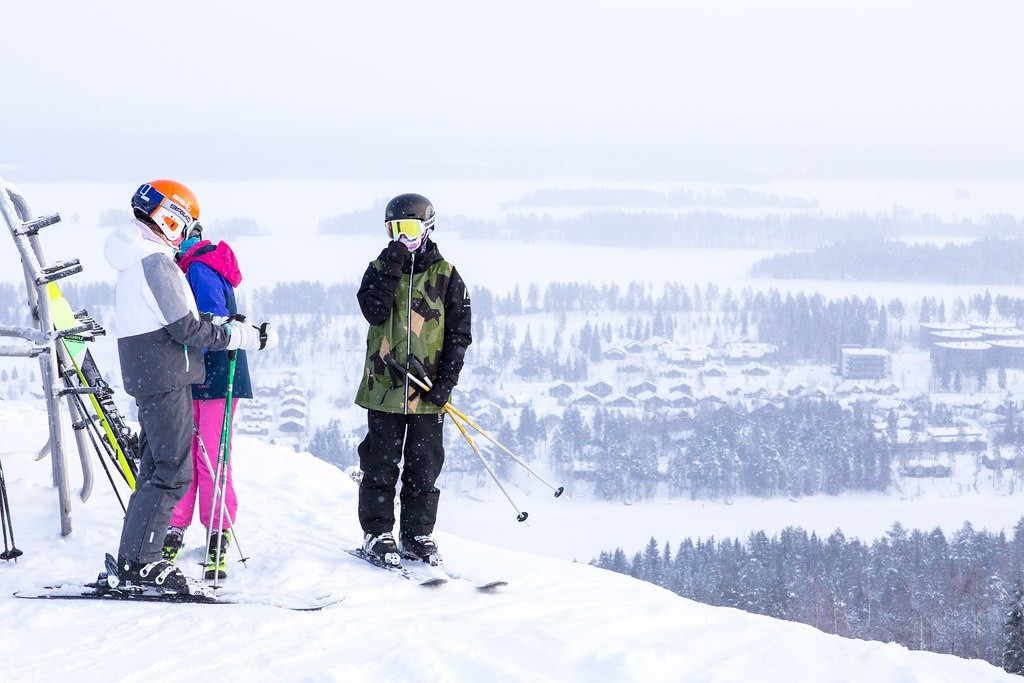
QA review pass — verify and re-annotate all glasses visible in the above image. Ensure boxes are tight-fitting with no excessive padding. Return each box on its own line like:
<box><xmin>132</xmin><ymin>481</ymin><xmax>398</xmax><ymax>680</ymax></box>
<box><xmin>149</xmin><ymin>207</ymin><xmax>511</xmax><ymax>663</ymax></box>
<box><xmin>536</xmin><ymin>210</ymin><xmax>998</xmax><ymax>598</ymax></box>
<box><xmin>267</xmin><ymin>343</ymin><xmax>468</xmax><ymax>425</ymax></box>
<box><xmin>382</xmin><ymin>219</ymin><xmax>425</xmax><ymax>242</ymax></box>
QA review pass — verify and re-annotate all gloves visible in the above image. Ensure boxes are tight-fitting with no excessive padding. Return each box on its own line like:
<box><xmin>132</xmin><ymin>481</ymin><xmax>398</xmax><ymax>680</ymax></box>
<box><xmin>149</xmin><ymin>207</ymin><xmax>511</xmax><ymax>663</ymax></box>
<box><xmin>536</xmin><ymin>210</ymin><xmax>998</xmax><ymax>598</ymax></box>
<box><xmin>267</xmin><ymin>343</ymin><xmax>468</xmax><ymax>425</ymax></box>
<box><xmin>212</xmin><ymin>313</ymin><xmax>281</xmax><ymax>353</ymax></box>
<box><xmin>381</xmin><ymin>239</ymin><xmax>408</xmax><ymax>278</ymax></box>
<box><xmin>419</xmin><ymin>380</ymin><xmax>451</xmax><ymax>407</ymax></box>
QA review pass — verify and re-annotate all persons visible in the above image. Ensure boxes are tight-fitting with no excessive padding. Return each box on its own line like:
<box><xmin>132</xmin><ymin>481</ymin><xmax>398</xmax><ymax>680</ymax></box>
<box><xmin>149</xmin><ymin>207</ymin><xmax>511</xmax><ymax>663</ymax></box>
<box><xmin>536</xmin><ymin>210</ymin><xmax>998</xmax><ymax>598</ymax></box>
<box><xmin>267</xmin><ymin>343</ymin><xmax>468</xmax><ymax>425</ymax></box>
<box><xmin>355</xmin><ymin>193</ymin><xmax>471</xmax><ymax>568</ymax></box>
<box><xmin>115</xmin><ymin>180</ymin><xmax>280</xmax><ymax>585</ymax></box>
<box><xmin>161</xmin><ymin>222</ymin><xmax>253</xmax><ymax>579</ymax></box>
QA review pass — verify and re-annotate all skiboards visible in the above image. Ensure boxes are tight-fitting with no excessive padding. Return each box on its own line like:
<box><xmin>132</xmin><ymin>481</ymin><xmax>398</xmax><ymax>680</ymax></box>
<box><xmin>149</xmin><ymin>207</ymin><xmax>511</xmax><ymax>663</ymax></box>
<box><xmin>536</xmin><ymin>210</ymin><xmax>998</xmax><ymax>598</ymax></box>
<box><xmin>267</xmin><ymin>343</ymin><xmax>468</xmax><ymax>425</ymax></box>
<box><xmin>342</xmin><ymin>536</ymin><xmax>510</xmax><ymax>590</ymax></box>
<box><xmin>12</xmin><ymin>578</ymin><xmax>345</xmax><ymax>608</ymax></box>
<box><xmin>159</xmin><ymin>530</ymin><xmax>232</xmax><ymax>578</ymax></box>
<box><xmin>33</xmin><ymin>253</ymin><xmax>148</xmax><ymax>499</ymax></box>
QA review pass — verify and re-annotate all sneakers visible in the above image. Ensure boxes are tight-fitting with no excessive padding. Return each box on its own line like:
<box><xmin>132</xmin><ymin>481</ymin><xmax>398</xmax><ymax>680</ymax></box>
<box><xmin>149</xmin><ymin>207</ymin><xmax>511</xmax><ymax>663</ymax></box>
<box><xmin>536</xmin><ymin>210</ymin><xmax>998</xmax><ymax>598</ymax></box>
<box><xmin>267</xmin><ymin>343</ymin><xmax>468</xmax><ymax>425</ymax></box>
<box><xmin>207</xmin><ymin>526</ymin><xmax>231</xmax><ymax>548</ymax></box>
<box><xmin>163</xmin><ymin>525</ymin><xmax>187</xmax><ymax>546</ymax></box>
<box><xmin>399</xmin><ymin>530</ymin><xmax>438</xmax><ymax>557</ymax></box>
<box><xmin>362</xmin><ymin>530</ymin><xmax>401</xmax><ymax>566</ymax></box>
<box><xmin>117</xmin><ymin>553</ymin><xmax>186</xmax><ymax>586</ymax></box>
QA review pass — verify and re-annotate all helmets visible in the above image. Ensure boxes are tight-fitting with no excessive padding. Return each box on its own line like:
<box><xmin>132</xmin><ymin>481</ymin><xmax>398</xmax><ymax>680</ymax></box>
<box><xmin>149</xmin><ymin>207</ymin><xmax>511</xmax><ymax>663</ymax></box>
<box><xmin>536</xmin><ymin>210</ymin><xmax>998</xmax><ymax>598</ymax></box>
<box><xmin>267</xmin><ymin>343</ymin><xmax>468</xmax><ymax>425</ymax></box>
<box><xmin>131</xmin><ymin>179</ymin><xmax>201</xmax><ymax>241</ymax></box>
<box><xmin>385</xmin><ymin>192</ymin><xmax>437</xmax><ymax>231</ymax></box>
